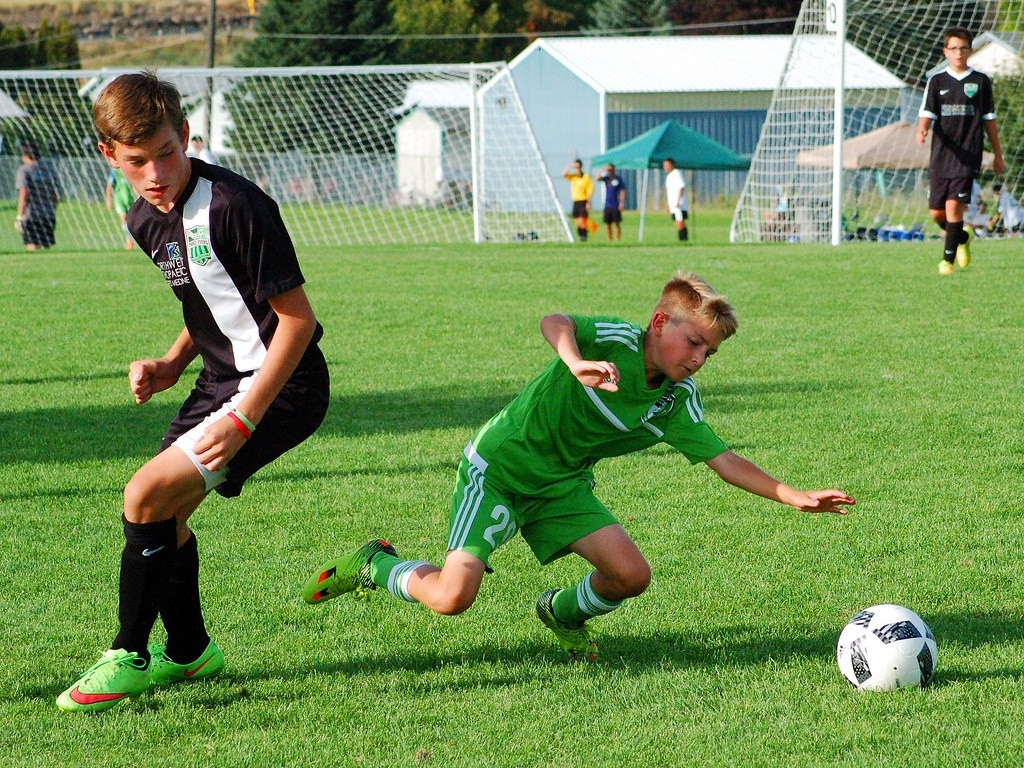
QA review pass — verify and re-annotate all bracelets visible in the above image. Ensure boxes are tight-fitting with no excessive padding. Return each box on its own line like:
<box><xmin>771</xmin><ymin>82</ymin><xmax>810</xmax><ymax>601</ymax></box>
<box><xmin>226</xmin><ymin>409</ymin><xmax>257</xmax><ymax>440</ymax></box>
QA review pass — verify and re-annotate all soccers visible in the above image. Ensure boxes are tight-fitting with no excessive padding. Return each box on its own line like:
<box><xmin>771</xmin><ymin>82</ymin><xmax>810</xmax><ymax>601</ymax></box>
<box><xmin>836</xmin><ymin>604</ymin><xmax>937</xmax><ymax>697</ymax></box>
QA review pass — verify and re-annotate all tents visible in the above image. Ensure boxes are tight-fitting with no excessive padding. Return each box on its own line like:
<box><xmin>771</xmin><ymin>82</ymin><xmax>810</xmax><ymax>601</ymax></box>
<box><xmin>795</xmin><ymin>120</ymin><xmax>1003</xmax><ymax>171</ymax></box>
<box><xmin>588</xmin><ymin>120</ymin><xmax>752</xmax><ymax>171</ymax></box>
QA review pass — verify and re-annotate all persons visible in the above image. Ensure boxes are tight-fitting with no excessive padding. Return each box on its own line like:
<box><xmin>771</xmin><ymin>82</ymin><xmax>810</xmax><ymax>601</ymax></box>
<box><xmin>302</xmin><ymin>273</ymin><xmax>856</xmax><ymax>661</ymax></box>
<box><xmin>963</xmin><ymin>179</ymin><xmax>1024</xmax><ymax>238</ymax></box>
<box><xmin>15</xmin><ymin>144</ymin><xmax>65</xmax><ymax>251</ymax></box>
<box><xmin>53</xmin><ymin>73</ymin><xmax>332</xmax><ymax>715</ymax></box>
<box><xmin>560</xmin><ymin>159</ymin><xmax>627</xmax><ymax>242</ymax></box>
<box><xmin>664</xmin><ymin>158</ymin><xmax>689</xmax><ymax>242</ymax></box>
<box><xmin>915</xmin><ymin>27</ymin><xmax>1004</xmax><ymax>275</ymax></box>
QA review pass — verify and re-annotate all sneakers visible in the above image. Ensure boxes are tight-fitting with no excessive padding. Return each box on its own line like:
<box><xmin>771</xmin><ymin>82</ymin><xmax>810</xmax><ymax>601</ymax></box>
<box><xmin>55</xmin><ymin>648</ymin><xmax>153</xmax><ymax>714</ymax></box>
<box><xmin>304</xmin><ymin>539</ymin><xmax>398</xmax><ymax>605</ymax></box>
<box><xmin>148</xmin><ymin>637</ymin><xmax>225</xmax><ymax>684</ymax></box>
<box><xmin>537</xmin><ymin>588</ymin><xmax>599</xmax><ymax>660</ymax></box>
<box><xmin>956</xmin><ymin>228</ymin><xmax>973</xmax><ymax>267</ymax></box>
<box><xmin>939</xmin><ymin>260</ymin><xmax>953</xmax><ymax>274</ymax></box>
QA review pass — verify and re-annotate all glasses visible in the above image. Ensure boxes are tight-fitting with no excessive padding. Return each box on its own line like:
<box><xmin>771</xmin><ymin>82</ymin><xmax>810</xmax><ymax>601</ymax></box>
<box><xmin>946</xmin><ymin>46</ymin><xmax>970</xmax><ymax>52</ymax></box>
